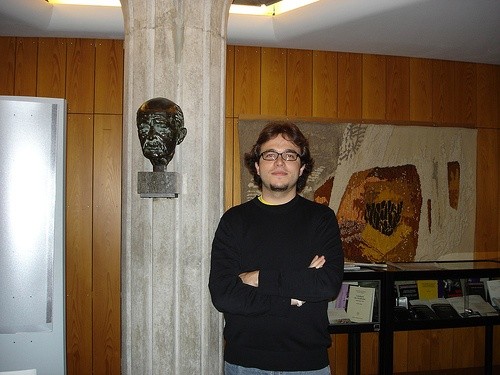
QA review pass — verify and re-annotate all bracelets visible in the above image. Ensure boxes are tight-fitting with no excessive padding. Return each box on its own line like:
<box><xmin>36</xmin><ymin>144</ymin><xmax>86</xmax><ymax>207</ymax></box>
<box><xmin>297</xmin><ymin>300</ymin><xmax>302</xmax><ymax>307</ymax></box>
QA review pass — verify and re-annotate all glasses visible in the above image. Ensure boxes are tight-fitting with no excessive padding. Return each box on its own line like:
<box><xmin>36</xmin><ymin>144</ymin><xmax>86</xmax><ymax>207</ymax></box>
<box><xmin>258</xmin><ymin>150</ymin><xmax>302</xmax><ymax>161</ymax></box>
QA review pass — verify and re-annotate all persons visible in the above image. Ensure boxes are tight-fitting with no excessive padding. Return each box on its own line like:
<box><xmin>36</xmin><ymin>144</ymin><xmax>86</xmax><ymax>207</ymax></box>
<box><xmin>207</xmin><ymin>122</ymin><xmax>344</xmax><ymax>375</ymax></box>
<box><xmin>136</xmin><ymin>97</ymin><xmax>188</xmax><ymax>167</ymax></box>
